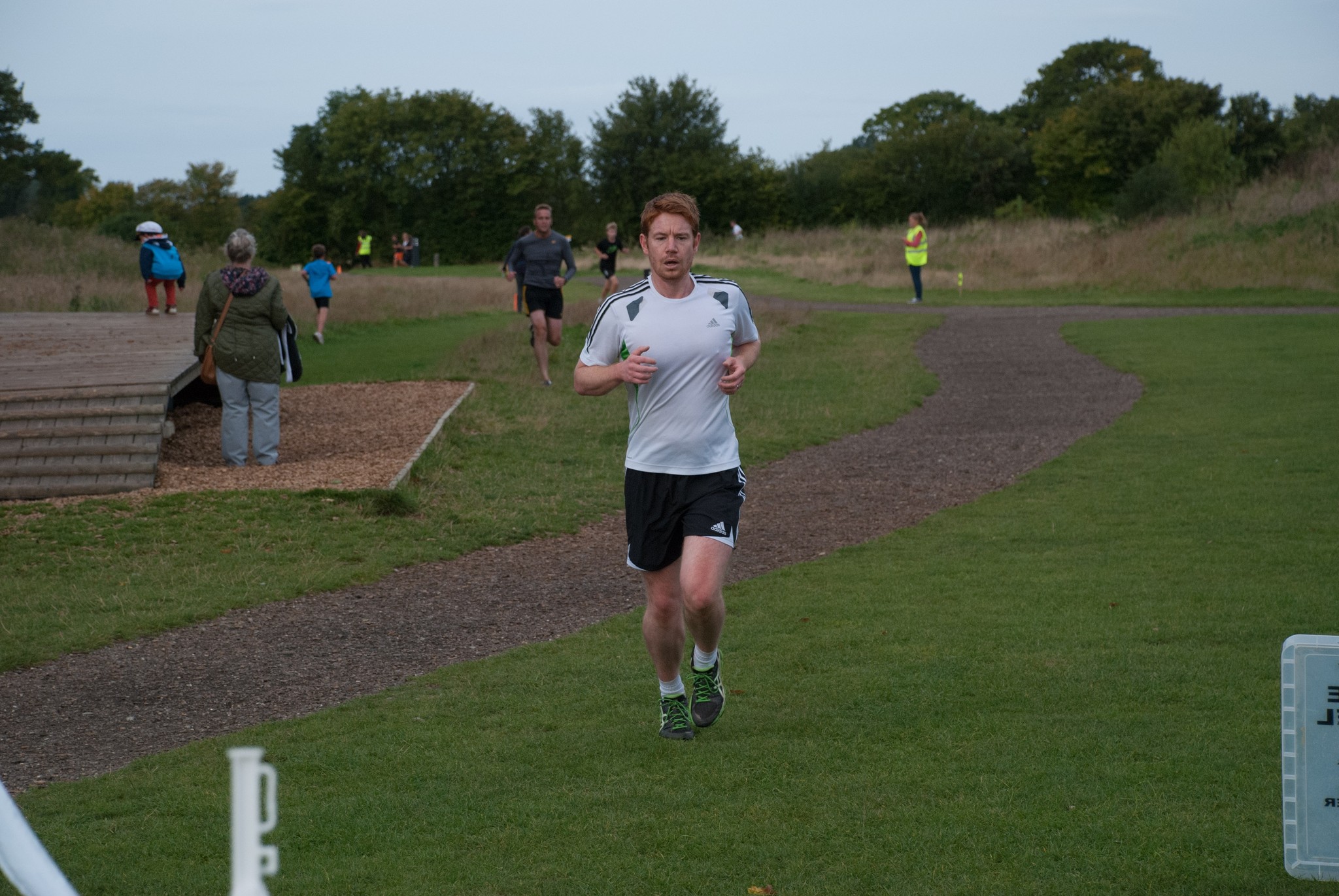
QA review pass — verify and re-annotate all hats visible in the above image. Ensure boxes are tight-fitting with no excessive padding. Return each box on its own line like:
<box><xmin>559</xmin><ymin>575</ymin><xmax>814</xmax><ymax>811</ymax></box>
<box><xmin>135</xmin><ymin>221</ymin><xmax>162</xmax><ymax>233</ymax></box>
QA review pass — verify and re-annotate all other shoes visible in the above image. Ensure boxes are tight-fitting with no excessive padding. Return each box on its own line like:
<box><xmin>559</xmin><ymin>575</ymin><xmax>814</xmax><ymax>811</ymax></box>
<box><xmin>544</xmin><ymin>380</ymin><xmax>552</xmax><ymax>385</ymax></box>
<box><xmin>165</xmin><ymin>305</ymin><xmax>177</xmax><ymax>314</ymax></box>
<box><xmin>146</xmin><ymin>307</ymin><xmax>160</xmax><ymax>315</ymax></box>
<box><xmin>529</xmin><ymin>326</ymin><xmax>534</xmax><ymax>347</ymax></box>
<box><xmin>313</xmin><ymin>332</ymin><xmax>324</xmax><ymax>345</ymax></box>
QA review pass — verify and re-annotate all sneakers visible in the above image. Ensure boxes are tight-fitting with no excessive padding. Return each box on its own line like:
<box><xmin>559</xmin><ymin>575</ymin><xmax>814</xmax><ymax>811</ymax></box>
<box><xmin>657</xmin><ymin>694</ymin><xmax>695</xmax><ymax>740</ymax></box>
<box><xmin>689</xmin><ymin>646</ymin><xmax>726</xmax><ymax>727</ymax></box>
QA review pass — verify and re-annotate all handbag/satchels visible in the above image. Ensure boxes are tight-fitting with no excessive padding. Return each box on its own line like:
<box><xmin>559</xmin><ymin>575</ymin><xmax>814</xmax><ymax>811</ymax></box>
<box><xmin>200</xmin><ymin>345</ymin><xmax>217</xmax><ymax>385</ymax></box>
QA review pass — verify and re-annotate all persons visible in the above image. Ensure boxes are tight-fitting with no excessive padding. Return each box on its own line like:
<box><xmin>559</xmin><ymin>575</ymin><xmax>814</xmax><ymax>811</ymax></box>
<box><xmin>136</xmin><ymin>217</ymin><xmax>412</xmax><ymax>345</ymax></box>
<box><xmin>195</xmin><ymin>227</ymin><xmax>288</xmax><ymax>464</ymax></box>
<box><xmin>900</xmin><ymin>211</ymin><xmax>930</xmax><ymax>302</ymax></box>
<box><xmin>504</xmin><ymin>203</ymin><xmax>744</xmax><ymax>387</ymax></box>
<box><xmin>573</xmin><ymin>195</ymin><xmax>761</xmax><ymax>741</ymax></box>
